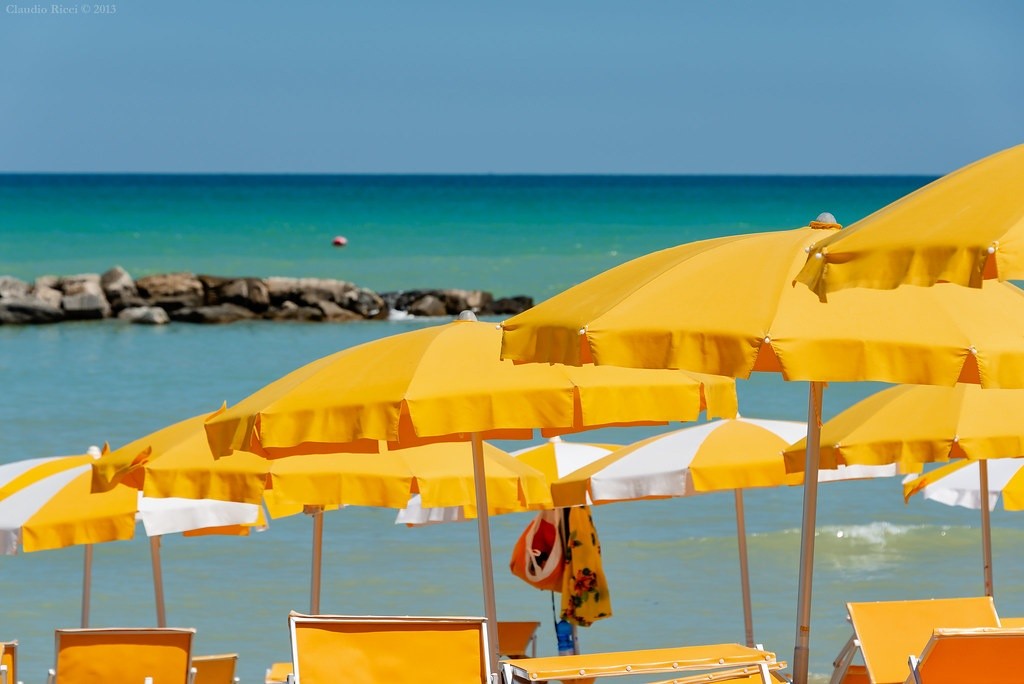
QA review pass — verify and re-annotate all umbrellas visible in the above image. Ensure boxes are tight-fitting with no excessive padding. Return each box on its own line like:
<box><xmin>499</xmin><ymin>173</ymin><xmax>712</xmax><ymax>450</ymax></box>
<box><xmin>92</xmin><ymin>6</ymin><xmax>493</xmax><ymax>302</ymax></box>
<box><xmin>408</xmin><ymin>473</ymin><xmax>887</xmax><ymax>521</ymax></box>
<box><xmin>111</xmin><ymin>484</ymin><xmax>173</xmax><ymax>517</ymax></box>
<box><xmin>0</xmin><ymin>144</ymin><xmax>1024</xmax><ymax>684</ymax></box>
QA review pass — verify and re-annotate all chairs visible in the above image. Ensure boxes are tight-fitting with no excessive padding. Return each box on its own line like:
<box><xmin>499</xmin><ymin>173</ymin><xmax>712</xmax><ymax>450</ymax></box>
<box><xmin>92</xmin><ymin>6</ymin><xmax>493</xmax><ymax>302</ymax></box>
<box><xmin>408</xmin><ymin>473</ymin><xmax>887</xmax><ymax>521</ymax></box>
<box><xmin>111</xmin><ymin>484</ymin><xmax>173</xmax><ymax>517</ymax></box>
<box><xmin>0</xmin><ymin>627</ymin><xmax>241</xmax><ymax>684</ymax></box>
<box><xmin>831</xmin><ymin>596</ymin><xmax>1024</xmax><ymax>684</ymax></box>
<box><xmin>265</xmin><ymin>611</ymin><xmax>790</xmax><ymax>684</ymax></box>
